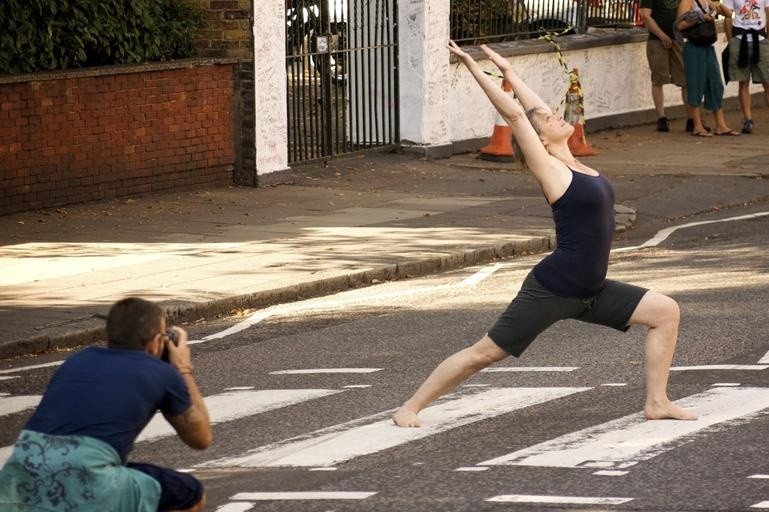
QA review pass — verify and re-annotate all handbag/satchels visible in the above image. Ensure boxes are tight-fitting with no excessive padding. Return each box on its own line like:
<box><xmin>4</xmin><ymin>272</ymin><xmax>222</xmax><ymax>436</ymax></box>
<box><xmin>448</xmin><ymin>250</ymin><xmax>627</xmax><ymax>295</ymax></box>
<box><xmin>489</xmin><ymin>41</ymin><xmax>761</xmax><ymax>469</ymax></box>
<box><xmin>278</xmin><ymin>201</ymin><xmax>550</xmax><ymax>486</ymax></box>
<box><xmin>681</xmin><ymin>0</ymin><xmax>718</xmax><ymax>46</ymax></box>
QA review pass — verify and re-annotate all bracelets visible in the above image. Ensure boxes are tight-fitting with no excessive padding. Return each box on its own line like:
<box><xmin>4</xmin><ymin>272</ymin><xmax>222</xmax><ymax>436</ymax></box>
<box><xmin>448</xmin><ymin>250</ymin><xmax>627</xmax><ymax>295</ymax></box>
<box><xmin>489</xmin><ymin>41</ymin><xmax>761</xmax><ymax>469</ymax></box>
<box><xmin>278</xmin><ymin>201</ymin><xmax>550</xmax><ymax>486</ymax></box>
<box><xmin>177</xmin><ymin>362</ymin><xmax>194</xmax><ymax>373</ymax></box>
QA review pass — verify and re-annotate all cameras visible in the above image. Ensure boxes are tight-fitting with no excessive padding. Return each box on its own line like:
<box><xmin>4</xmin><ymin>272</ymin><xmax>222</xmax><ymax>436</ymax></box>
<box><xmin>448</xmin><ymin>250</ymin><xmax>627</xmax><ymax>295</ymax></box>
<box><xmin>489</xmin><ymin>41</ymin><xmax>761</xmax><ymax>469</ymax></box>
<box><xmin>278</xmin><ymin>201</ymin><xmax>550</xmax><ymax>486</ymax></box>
<box><xmin>161</xmin><ymin>327</ymin><xmax>181</xmax><ymax>365</ymax></box>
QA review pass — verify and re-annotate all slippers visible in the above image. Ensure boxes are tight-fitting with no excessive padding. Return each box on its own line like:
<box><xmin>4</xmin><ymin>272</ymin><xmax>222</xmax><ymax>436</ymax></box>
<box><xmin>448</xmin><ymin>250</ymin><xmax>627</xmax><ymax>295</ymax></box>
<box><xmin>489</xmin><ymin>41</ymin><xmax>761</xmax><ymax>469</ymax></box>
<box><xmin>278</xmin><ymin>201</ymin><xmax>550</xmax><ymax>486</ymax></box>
<box><xmin>690</xmin><ymin>130</ymin><xmax>713</xmax><ymax>137</ymax></box>
<box><xmin>714</xmin><ymin>128</ymin><xmax>742</xmax><ymax>137</ymax></box>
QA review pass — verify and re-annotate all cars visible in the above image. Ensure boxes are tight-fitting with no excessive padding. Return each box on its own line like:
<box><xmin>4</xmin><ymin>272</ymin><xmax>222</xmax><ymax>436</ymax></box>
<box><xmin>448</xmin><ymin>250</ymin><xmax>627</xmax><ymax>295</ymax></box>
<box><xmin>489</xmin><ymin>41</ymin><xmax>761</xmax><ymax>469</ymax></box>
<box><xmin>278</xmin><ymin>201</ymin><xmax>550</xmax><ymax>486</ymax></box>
<box><xmin>299</xmin><ymin>4</ymin><xmax>576</xmax><ymax>87</ymax></box>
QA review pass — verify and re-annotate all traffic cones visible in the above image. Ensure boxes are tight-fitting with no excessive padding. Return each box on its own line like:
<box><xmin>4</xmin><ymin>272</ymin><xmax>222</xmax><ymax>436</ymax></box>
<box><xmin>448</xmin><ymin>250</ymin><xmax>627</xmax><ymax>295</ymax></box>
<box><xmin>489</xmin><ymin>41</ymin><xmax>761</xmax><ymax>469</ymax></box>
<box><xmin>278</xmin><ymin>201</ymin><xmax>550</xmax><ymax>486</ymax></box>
<box><xmin>475</xmin><ymin>77</ymin><xmax>517</xmax><ymax>163</ymax></box>
<box><xmin>564</xmin><ymin>68</ymin><xmax>598</xmax><ymax>156</ymax></box>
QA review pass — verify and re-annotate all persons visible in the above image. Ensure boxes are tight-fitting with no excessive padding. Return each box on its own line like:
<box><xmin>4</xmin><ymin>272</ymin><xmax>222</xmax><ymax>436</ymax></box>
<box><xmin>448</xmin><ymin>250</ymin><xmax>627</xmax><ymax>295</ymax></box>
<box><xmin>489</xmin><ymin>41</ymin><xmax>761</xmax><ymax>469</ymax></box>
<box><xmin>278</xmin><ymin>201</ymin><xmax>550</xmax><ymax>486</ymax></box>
<box><xmin>391</xmin><ymin>35</ymin><xmax>703</xmax><ymax>428</ymax></box>
<box><xmin>724</xmin><ymin>0</ymin><xmax>769</xmax><ymax>133</ymax></box>
<box><xmin>672</xmin><ymin>0</ymin><xmax>741</xmax><ymax>137</ymax></box>
<box><xmin>637</xmin><ymin>0</ymin><xmax>710</xmax><ymax>133</ymax></box>
<box><xmin>0</xmin><ymin>298</ymin><xmax>218</xmax><ymax>511</ymax></box>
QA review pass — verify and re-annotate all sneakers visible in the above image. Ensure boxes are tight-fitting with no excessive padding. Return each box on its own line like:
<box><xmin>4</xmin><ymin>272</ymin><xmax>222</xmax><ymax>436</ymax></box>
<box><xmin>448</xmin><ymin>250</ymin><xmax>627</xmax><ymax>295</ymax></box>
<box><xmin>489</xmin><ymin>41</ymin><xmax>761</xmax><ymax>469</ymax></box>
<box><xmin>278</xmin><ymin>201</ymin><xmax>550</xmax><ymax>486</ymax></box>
<box><xmin>656</xmin><ymin>116</ymin><xmax>670</xmax><ymax>132</ymax></box>
<box><xmin>741</xmin><ymin>119</ymin><xmax>753</xmax><ymax>134</ymax></box>
<box><xmin>685</xmin><ymin>118</ymin><xmax>711</xmax><ymax>132</ymax></box>
